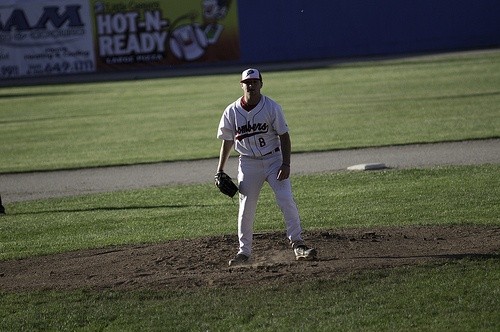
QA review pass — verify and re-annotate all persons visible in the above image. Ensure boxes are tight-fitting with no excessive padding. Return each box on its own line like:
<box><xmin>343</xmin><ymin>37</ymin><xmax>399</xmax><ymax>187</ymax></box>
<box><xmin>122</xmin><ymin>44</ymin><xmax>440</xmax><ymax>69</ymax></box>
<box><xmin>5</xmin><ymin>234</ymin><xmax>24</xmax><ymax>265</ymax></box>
<box><xmin>214</xmin><ymin>68</ymin><xmax>318</xmax><ymax>265</ymax></box>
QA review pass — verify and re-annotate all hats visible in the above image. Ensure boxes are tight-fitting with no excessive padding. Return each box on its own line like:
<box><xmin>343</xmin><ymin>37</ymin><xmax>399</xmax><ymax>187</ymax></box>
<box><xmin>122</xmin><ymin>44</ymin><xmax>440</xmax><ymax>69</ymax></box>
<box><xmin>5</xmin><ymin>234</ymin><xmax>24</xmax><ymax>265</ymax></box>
<box><xmin>240</xmin><ymin>68</ymin><xmax>262</xmax><ymax>81</ymax></box>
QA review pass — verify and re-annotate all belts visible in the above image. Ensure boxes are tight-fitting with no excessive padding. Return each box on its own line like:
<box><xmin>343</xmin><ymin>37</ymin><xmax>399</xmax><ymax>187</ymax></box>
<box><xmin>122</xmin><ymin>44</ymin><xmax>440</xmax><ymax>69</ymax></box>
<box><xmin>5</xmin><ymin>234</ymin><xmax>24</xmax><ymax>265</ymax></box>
<box><xmin>263</xmin><ymin>147</ymin><xmax>280</xmax><ymax>156</ymax></box>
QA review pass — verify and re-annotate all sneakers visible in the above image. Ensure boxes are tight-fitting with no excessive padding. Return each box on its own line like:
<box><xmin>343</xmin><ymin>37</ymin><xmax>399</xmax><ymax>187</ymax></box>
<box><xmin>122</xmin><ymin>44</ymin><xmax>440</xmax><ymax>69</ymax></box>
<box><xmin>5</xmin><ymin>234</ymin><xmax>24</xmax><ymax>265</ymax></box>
<box><xmin>294</xmin><ymin>245</ymin><xmax>316</xmax><ymax>259</ymax></box>
<box><xmin>229</xmin><ymin>254</ymin><xmax>248</xmax><ymax>266</ymax></box>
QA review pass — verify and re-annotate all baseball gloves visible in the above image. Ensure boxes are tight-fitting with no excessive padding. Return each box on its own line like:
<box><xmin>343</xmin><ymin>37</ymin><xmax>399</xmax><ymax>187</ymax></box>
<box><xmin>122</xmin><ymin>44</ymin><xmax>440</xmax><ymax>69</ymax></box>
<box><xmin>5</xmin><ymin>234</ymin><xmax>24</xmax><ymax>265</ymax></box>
<box><xmin>214</xmin><ymin>172</ymin><xmax>239</xmax><ymax>199</ymax></box>
